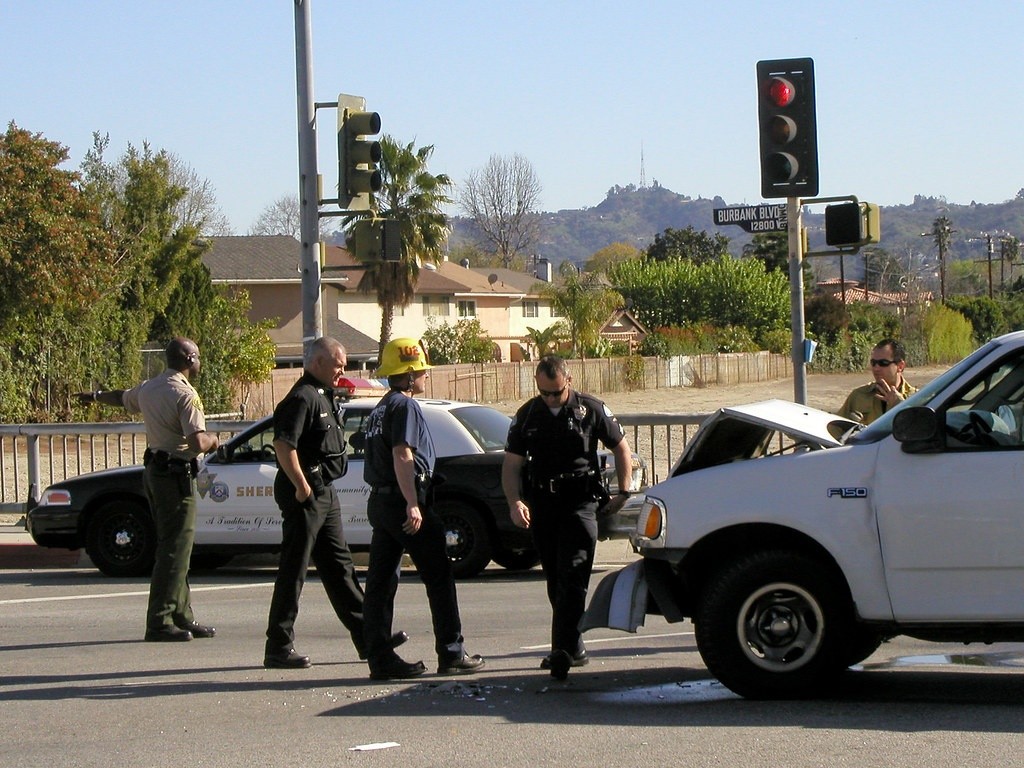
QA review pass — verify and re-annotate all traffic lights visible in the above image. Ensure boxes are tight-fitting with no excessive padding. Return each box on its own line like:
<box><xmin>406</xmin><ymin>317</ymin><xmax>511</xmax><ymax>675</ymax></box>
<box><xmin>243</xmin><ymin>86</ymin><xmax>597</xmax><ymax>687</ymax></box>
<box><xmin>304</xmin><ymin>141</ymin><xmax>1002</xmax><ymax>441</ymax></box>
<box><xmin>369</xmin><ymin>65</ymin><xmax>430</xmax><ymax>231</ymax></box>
<box><xmin>337</xmin><ymin>91</ymin><xmax>383</xmax><ymax>212</ymax></box>
<box><xmin>755</xmin><ymin>56</ymin><xmax>821</xmax><ymax>198</ymax></box>
<box><xmin>824</xmin><ymin>201</ymin><xmax>882</xmax><ymax>248</ymax></box>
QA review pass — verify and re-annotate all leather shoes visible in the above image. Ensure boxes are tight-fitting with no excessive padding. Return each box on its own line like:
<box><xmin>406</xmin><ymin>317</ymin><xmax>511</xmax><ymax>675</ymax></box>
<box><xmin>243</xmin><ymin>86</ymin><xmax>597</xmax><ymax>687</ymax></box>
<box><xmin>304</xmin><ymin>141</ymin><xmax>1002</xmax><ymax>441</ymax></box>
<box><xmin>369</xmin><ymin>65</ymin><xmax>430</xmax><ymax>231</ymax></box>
<box><xmin>145</xmin><ymin>625</ymin><xmax>193</xmax><ymax>641</ymax></box>
<box><xmin>551</xmin><ymin>650</ymin><xmax>571</xmax><ymax>681</ymax></box>
<box><xmin>356</xmin><ymin>630</ymin><xmax>409</xmax><ymax>660</ymax></box>
<box><xmin>178</xmin><ymin>619</ymin><xmax>216</xmax><ymax>637</ymax></box>
<box><xmin>368</xmin><ymin>657</ymin><xmax>428</xmax><ymax>681</ymax></box>
<box><xmin>540</xmin><ymin>648</ymin><xmax>588</xmax><ymax>670</ymax></box>
<box><xmin>264</xmin><ymin>649</ymin><xmax>311</xmax><ymax>668</ymax></box>
<box><xmin>437</xmin><ymin>652</ymin><xmax>485</xmax><ymax>676</ymax></box>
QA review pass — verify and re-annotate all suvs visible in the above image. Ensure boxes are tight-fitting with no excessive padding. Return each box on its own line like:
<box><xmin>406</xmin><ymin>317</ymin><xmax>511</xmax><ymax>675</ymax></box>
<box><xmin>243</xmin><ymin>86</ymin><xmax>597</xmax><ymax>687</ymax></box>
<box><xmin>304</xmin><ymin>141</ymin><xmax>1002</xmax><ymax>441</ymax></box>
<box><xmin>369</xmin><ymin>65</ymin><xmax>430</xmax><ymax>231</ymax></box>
<box><xmin>624</xmin><ymin>330</ymin><xmax>1024</xmax><ymax>702</ymax></box>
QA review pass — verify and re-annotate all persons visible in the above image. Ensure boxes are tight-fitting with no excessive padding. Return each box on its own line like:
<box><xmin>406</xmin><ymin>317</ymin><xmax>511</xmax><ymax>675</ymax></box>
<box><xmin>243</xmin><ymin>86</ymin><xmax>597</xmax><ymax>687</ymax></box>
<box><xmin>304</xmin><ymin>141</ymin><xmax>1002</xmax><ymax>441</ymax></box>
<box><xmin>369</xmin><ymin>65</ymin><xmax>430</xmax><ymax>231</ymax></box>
<box><xmin>73</xmin><ymin>338</ymin><xmax>218</xmax><ymax>641</ymax></box>
<box><xmin>502</xmin><ymin>356</ymin><xmax>632</xmax><ymax>680</ymax></box>
<box><xmin>363</xmin><ymin>338</ymin><xmax>485</xmax><ymax>681</ymax></box>
<box><xmin>264</xmin><ymin>337</ymin><xmax>409</xmax><ymax>668</ymax></box>
<box><xmin>828</xmin><ymin>339</ymin><xmax>919</xmax><ymax>443</ymax></box>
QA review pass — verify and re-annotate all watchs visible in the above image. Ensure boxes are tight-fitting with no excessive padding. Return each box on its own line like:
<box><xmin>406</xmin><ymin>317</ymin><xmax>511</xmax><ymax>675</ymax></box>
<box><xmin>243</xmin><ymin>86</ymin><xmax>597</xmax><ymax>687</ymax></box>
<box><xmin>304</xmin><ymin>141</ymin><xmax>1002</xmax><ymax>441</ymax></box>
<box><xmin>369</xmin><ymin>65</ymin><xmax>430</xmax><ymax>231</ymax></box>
<box><xmin>619</xmin><ymin>490</ymin><xmax>630</xmax><ymax>498</ymax></box>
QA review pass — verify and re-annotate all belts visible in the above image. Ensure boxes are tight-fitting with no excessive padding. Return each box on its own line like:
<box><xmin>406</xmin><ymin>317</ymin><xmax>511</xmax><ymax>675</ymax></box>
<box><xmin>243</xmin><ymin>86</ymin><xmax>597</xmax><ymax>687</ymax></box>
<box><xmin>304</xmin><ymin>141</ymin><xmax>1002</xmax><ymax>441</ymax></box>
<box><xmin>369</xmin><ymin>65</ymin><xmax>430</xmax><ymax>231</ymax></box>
<box><xmin>323</xmin><ymin>478</ymin><xmax>332</xmax><ymax>486</ymax></box>
<box><xmin>373</xmin><ymin>487</ymin><xmax>402</xmax><ymax>494</ymax></box>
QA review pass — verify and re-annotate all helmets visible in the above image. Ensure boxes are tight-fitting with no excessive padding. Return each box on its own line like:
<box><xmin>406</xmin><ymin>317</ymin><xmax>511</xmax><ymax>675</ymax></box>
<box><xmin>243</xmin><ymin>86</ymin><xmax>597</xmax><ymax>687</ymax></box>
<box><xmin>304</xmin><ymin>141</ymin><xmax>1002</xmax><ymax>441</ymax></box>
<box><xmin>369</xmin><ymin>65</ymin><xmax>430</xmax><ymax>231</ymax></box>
<box><xmin>370</xmin><ymin>338</ymin><xmax>435</xmax><ymax>377</ymax></box>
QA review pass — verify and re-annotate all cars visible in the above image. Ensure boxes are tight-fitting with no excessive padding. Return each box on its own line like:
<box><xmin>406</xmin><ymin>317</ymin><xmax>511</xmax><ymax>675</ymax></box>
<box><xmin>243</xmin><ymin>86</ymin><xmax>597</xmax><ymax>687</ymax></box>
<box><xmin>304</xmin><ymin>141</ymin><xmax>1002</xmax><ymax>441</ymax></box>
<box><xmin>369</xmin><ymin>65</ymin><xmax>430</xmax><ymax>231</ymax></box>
<box><xmin>24</xmin><ymin>376</ymin><xmax>650</xmax><ymax>583</ymax></box>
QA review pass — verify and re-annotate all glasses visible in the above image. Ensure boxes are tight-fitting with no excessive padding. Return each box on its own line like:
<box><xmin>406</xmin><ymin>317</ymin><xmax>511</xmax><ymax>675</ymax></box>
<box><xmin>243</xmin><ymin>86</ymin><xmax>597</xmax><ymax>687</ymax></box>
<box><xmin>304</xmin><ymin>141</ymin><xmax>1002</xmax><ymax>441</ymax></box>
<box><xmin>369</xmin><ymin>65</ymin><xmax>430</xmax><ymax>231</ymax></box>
<box><xmin>870</xmin><ymin>359</ymin><xmax>898</xmax><ymax>367</ymax></box>
<box><xmin>538</xmin><ymin>382</ymin><xmax>568</xmax><ymax>397</ymax></box>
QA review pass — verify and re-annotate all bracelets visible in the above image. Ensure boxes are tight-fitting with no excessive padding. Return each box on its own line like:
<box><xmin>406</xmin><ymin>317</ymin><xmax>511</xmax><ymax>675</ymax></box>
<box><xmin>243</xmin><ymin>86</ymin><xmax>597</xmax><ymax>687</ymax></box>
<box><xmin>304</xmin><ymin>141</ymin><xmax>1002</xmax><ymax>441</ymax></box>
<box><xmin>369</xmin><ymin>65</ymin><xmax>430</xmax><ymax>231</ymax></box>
<box><xmin>94</xmin><ymin>390</ymin><xmax>102</xmax><ymax>404</ymax></box>
